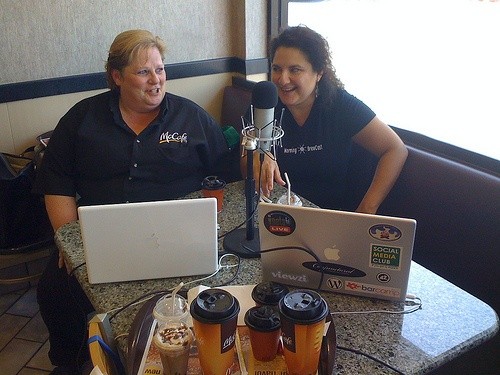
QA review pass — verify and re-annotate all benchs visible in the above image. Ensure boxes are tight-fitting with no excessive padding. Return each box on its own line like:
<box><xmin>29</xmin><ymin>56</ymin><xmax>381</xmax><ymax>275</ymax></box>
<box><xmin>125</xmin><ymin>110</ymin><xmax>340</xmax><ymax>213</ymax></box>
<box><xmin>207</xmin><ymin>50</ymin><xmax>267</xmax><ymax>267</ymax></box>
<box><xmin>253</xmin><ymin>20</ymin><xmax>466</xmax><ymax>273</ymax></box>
<box><xmin>219</xmin><ymin>85</ymin><xmax>500</xmax><ymax>375</ymax></box>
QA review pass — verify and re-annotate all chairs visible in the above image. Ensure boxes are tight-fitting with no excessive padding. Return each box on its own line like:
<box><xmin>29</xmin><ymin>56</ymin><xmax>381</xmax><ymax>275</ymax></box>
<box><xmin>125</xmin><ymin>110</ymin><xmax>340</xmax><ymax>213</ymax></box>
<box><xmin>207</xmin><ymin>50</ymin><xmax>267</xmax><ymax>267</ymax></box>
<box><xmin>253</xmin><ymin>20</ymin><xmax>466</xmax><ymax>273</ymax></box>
<box><xmin>88</xmin><ymin>313</ymin><xmax>126</xmax><ymax>375</ymax></box>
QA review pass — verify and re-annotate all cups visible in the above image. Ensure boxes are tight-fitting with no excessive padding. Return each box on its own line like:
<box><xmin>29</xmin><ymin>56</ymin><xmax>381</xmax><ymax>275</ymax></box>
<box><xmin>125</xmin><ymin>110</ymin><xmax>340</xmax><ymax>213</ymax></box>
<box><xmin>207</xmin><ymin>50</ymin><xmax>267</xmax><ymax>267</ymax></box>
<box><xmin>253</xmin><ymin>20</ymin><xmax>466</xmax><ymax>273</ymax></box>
<box><xmin>244</xmin><ymin>305</ymin><xmax>282</xmax><ymax>362</ymax></box>
<box><xmin>277</xmin><ymin>192</ymin><xmax>303</xmax><ymax>208</ymax></box>
<box><xmin>154</xmin><ymin>320</ymin><xmax>193</xmax><ymax>375</ymax></box>
<box><xmin>190</xmin><ymin>288</ymin><xmax>240</xmax><ymax>375</ymax></box>
<box><xmin>152</xmin><ymin>294</ymin><xmax>189</xmax><ymax>329</ymax></box>
<box><xmin>201</xmin><ymin>176</ymin><xmax>226</xmax><ymax>211</ymax></box>
<box><xmin>279</xmin><ymin>289</ymin><xmax>329</xmax><ymax>375</ymax></box>
<box><xmin>252</xmin><ymin>281</ymin><xmax>289</xmax><ymax>309</ymax></box>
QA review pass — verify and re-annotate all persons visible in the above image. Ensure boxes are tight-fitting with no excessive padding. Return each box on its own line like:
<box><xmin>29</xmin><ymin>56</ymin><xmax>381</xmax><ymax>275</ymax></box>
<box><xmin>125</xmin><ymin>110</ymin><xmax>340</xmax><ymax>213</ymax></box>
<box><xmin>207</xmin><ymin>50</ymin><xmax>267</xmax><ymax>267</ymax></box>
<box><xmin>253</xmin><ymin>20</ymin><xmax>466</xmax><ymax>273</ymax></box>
<box><xmin>35</xmin><ymin>30</ymin><xmax>238</xmax><ymax>375</ymax></box>
<box><xmin>239</xmin><ymin>25</ymin><xmax>408</xmax><ymax>214</ymax></box>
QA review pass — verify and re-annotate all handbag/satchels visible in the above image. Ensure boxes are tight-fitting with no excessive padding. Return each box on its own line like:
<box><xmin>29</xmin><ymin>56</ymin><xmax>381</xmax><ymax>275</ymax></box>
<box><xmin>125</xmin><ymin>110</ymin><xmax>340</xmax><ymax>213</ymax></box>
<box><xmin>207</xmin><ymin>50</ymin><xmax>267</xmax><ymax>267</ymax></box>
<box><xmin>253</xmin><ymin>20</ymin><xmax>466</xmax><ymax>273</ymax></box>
<box><xmin>0</xmin><ymin>152</ymin><xmax>37</xmax><ymax>192</ymax></box>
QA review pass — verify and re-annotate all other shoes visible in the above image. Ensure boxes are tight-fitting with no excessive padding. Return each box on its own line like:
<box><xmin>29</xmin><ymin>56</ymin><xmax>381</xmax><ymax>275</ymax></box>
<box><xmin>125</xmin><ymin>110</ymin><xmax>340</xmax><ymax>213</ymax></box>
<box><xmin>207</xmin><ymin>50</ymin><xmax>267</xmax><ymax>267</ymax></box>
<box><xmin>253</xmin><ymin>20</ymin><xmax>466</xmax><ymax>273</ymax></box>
<box><xmin>49</xmin><ymin>362</ymin><xmax>88</xmax><ymax>375</ymax></box>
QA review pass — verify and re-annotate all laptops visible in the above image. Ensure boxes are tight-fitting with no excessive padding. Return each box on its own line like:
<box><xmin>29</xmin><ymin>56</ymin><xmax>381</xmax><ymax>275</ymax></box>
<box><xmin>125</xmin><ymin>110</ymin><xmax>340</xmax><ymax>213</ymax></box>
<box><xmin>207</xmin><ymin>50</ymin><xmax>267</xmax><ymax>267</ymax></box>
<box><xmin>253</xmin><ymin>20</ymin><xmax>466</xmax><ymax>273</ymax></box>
<box><xmin>78</xmin><ymin>197</ymin><xmax>218</xmax><ymax>284</ymax></box>
<box><xmin>257</xmin><ymin>202</ymin><xmax>417</xmax><ymax>302</ymax></box>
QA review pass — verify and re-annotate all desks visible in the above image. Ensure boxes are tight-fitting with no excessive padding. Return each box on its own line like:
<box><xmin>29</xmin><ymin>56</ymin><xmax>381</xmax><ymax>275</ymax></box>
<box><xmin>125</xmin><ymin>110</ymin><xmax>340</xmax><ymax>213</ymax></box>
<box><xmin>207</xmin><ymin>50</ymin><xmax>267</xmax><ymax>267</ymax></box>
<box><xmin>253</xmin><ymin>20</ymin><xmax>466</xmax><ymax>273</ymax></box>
<box><xmin>54</xmin><ymin>177</ymin><xmax>499</xmax><ymax>375</ymax></box>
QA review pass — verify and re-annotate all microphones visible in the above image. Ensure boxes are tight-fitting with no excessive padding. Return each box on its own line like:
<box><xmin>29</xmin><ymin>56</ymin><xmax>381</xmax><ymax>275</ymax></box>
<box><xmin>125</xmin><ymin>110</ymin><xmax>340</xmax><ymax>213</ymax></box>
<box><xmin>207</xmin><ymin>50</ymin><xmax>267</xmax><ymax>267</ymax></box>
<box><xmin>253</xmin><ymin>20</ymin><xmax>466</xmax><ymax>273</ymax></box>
<box><xmin>253</xmin><ymin>81</ymin><xmax>278</xmax><ymax>161</ymax></box>
